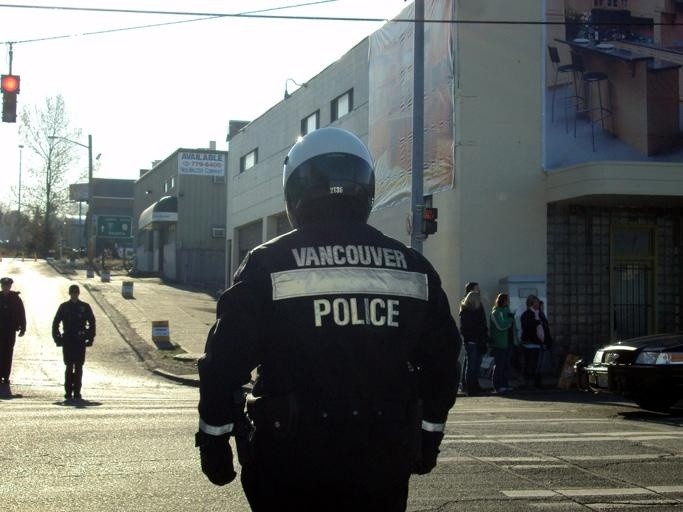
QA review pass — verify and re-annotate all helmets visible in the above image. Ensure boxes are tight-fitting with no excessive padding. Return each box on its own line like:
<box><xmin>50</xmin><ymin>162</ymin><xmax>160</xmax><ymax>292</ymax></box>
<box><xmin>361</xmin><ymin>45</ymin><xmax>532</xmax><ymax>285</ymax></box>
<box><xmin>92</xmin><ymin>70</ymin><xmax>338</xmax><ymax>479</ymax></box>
<box><xmin>283</xmin><ymin>128</ymin><xmax>376</xmax><ymax>227</ymax></box>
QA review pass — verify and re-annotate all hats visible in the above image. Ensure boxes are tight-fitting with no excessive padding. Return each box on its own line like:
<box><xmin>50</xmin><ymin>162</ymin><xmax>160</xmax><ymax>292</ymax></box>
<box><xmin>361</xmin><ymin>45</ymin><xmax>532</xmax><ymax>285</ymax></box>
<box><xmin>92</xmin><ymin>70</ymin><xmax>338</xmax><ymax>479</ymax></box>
<box><xmin>0</xmin><ymin>278</ymin><xmax>13</xmax><ymax>284</ymax></box>
<box><xmin>70</xmin><ymin>286</ymin><xmax>80</xmax><ymax>294</ymax></box>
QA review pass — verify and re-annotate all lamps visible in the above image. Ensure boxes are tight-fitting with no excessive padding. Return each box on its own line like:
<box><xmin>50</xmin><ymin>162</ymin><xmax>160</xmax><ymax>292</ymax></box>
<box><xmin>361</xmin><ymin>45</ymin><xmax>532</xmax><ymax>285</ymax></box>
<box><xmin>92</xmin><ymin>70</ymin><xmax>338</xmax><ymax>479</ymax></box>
<box><xmin>284</xmin><ymin>77</ymin><xmax>306</xmax><ymax>98</ymax></box>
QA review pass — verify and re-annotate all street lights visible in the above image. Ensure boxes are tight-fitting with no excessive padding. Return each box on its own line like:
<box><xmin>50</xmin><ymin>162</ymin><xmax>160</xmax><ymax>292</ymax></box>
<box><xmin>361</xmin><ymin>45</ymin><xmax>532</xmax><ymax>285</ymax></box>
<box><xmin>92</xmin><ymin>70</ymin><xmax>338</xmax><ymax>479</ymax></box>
<box><xmin>48</xmin><ymin>135</ymin><xmax>92</xmax><ymax>210</ymax></box>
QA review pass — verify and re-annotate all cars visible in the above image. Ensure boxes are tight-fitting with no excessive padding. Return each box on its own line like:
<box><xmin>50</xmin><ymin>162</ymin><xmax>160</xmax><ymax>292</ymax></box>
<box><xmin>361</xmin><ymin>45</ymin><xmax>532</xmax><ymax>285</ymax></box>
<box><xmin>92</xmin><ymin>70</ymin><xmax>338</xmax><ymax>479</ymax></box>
<box><xmin>72</xmin><ymin>246</ymin><xmax>87</xmax><ymax>259</ymax></box>
<box><xmin>582</xmin><ymin>331</ymin><xmax>683</xmax><ymax>415</ymax></box>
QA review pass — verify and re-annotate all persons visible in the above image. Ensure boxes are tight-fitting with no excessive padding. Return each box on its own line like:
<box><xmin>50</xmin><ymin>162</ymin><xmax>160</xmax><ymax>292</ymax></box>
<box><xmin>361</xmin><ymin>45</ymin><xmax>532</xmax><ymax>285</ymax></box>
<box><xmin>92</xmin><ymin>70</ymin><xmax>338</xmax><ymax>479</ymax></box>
<box><xmin>0</xmin><ymin>277</ymin><xmax>26</xmax><ymax>385</ymax></box>
<box><xmin>194</xmin><ymin>126</ymin><xmax>464</xmax><ymax>511</ymax></box>
<box><xmin>52</xmin><ymin>285</ymin><xmax>96</xmax><ymax>406</ymax></box>
<box><xmin>459</xmin><ymin>280</ymin><xmax>551</xmax><ymax>398</ymax></box>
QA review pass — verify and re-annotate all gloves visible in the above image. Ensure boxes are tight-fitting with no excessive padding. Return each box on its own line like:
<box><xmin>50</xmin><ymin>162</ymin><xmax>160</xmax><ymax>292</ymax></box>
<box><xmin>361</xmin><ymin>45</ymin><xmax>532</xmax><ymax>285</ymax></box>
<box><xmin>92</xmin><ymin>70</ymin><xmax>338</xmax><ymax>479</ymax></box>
<box><xmin>195</xmin><ymin>433</ymin><xmax>236</xmax><ymax>486</ymax></box>
<box><xmin>413</xmin><ymin>429</ymin><xmax>443</xmax><ymax>475</ymax></box>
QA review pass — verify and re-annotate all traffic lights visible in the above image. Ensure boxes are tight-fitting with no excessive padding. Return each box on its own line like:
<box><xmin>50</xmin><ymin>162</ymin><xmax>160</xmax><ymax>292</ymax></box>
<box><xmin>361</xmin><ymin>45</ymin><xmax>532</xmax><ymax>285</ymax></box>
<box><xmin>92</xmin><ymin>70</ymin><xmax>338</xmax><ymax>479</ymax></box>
<box><xmin>423</xmin><ymin>194</ymin><xmax>437</xmax><ymax>234</ymax></box>
<box><xmin>0</xmin><ymin>74</ymin><xmax>20</xmax><ymax>123</ymax></box>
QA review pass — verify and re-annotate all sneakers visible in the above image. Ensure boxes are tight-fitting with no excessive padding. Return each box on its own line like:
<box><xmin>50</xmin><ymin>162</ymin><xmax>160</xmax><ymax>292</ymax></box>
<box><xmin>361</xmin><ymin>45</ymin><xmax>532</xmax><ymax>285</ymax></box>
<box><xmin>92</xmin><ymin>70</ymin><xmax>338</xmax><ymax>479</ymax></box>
<box><xmin>461</xmin><ymin>382</ymin><xmax>549</xmax><ymax>394</ymax></box>
<box><xmin>66</xmin><ymin>398</ymin><xmax>82</xmax><ymax>404</ymax></box>
